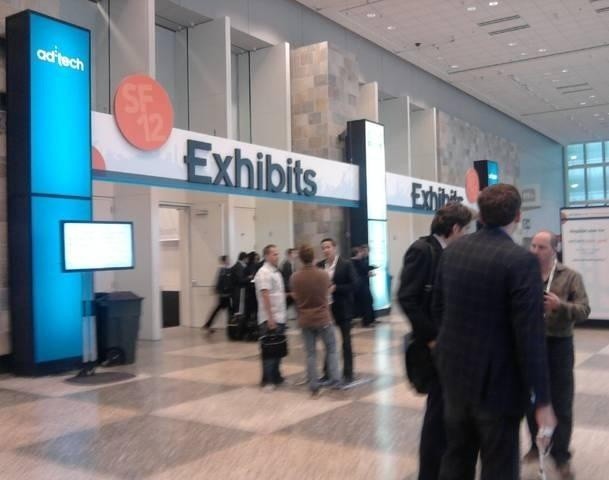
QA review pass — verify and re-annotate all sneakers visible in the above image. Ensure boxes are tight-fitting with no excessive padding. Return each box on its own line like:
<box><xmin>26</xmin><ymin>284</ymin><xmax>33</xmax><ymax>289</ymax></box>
<box><xmin>523</xmin><ymin>444</ymin><xmax>549</xmax><ymax>463</ymax></box>
<box><xmin>557</xmin><ymin>464</ymin><xmax>576</xmax><ymax>480</ymax></box>
<box><xmin>361</xmin><ymin>322</ymin><xmax>376</xmax><ymax>328</ymax></box>
<box><xmin>260</xmin><ymin>376</ymin><xmax>357</xmax><ymax>399</ymax></box>
<box><xmin>370</xmin><ymin>319</ymin><xmax>380</xmax><ymax>324</ymax></box>
<box><xmin>202</xmin><ymin>326</ymin><xmax>215</xmax><ymax>333</ymax></box>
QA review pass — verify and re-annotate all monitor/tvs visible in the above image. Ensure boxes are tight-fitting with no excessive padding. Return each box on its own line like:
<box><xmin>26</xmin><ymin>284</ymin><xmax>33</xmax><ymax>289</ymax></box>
<box><xmin>59</xmin><ymin>220</ymin><xmax>135</xmax><ymax>273</ymax></box>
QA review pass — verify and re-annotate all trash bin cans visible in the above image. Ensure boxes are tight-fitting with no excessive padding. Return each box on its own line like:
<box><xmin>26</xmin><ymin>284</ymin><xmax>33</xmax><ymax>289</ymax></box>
<box><xmin>95</xmin><ymin>291</ymin><xmax>144</xmax><ymax>366</ymax></box>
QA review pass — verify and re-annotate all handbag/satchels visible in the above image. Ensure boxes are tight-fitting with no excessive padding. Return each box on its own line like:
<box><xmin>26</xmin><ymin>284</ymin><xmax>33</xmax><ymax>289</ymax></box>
<box><xmin>259</xmin><ymin>334</ymin><xmax>288</xmax><ymax>359</ymax></box>
<box><xmin>402</xmin><ymin>333</ymin><xmax>434</xmax><ymax>393</ymax></box>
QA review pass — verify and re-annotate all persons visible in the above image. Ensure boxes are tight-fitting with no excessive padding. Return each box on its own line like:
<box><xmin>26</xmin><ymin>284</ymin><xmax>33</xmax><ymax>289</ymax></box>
<box><xmin>398</xmin><ymin>202</ymin><xmax>477</xmax><ymax>480</ymax></box>
<box><xmin>429</xmin><ymin>182</ymin><xmax>544</xmax><ymax>479</ymax></box>
<box><xmin>202</xmin><ymin>238</ymin><xmax>382</xmax><ymax>398</ymax></box>
